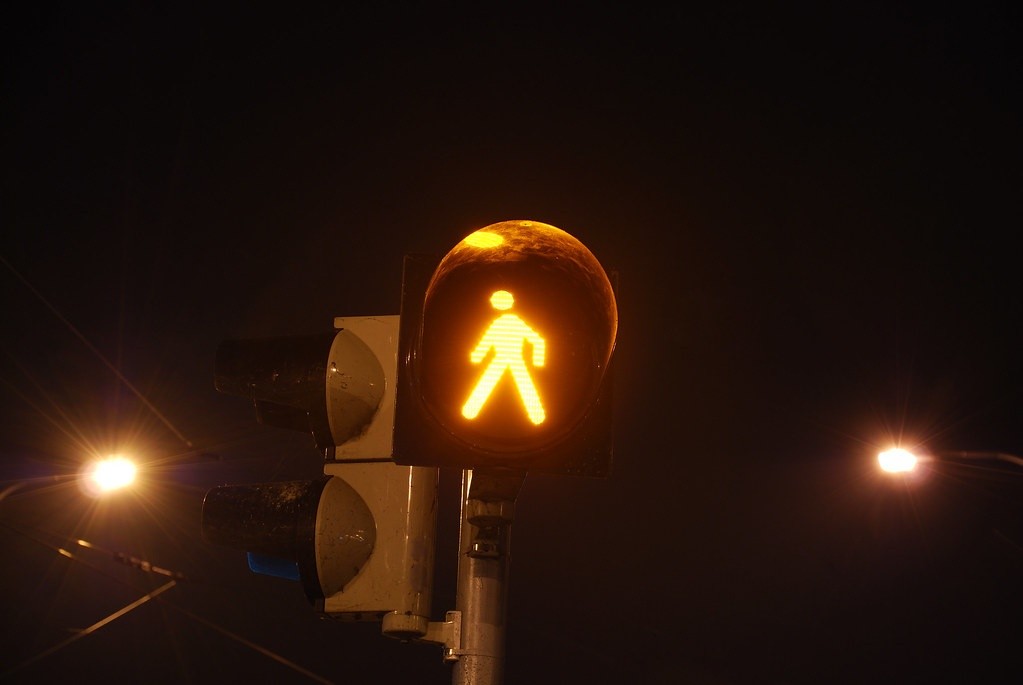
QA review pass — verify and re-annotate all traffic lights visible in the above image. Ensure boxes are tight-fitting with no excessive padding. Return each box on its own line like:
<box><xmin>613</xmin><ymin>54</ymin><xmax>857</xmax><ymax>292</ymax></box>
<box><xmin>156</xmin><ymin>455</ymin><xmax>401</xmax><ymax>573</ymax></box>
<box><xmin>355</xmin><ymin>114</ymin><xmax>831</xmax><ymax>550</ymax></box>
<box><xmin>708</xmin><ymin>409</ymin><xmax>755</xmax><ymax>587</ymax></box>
<box><xmin>199</xmin><ymin>312</ymin><xmax>442</xmax><ymax>644</ymax></box>
<box><xmin>391</xmin><ymin>218</ymin><xmax>620</xmax><ymax>480</ymax></box>
